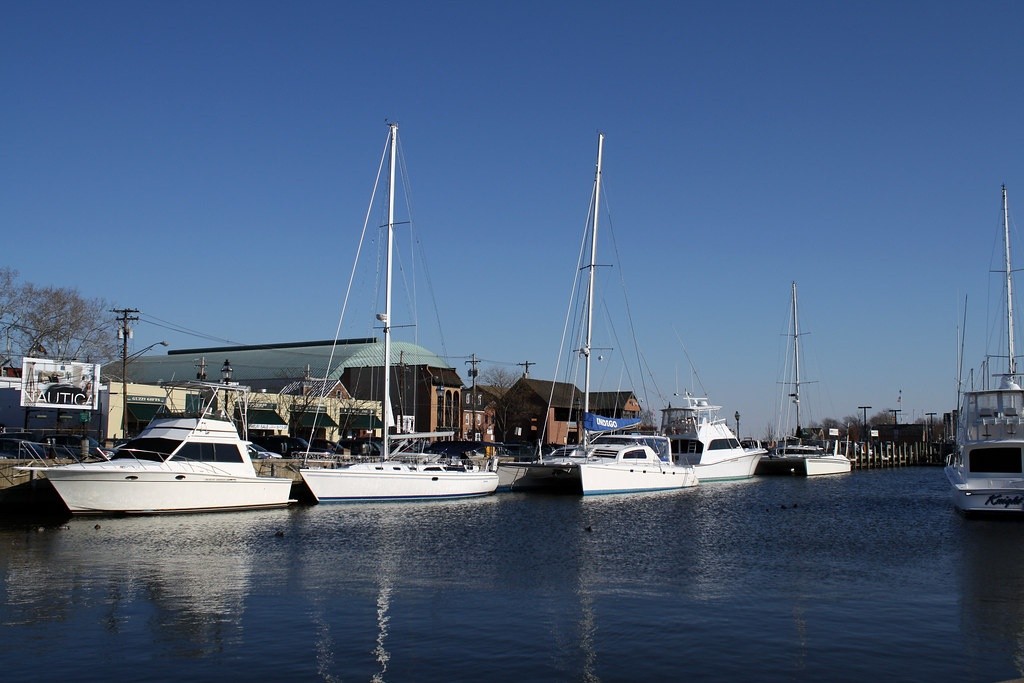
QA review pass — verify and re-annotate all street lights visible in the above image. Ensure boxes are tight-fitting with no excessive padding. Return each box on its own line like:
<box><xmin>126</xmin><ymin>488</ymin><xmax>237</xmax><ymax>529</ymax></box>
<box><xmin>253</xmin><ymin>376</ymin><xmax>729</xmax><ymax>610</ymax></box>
<box><xmin>735</xmin><ymin>412</ymin><xmax>740</xmax><ymax>443</ymax></box>
<box><xmin>925</xmin><ymin>413</ymin><xmax>938</xmax><ymax>441</ymax></box>
<box><xmin>859</xmin><ymin>406</ymin><xmax>872</xmax><ymax>443</ymax></box>
<box><xmin>889</xmin><ymin>409</ymin><xmax>901</xmax><ymax>441</ymax></box>
<box><xmin>221</xmin><ymin>359</ymin><xmax>234</xmax><ymax>416</ymax></box>
<box><xmin>667</xmin><ymin>401</ymin><xmax>673</xmax><ymax>428</ymax></box>
<box><xmin>435</xmin><ymin>383</ymin><xmax>446</xmax><ymax>454</ymax></box>
<box><xmin>123</xmin><ymin>340</ymin><xmax>169</xmax><ymax>438</ymax></box>
<box><xmin>573</xmin><ymin>396</ymin><xmax>581</xmax><ymax>444</ymax></box>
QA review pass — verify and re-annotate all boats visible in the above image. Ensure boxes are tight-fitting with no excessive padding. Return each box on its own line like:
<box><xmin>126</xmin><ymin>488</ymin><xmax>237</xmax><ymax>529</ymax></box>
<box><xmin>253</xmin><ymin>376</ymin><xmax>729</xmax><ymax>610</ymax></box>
<box><xmin>664</xmin><ymin>388</ymin><xmax>770</xmax><ymax>481</ymax></box>
<box><xmin>579</xmin><ymin>445</ymin><xmax>698</xmax><ymax>497</ymax></box>
<box><xmin>14</xmin><ymin>379</ymin><xmax>300</xmax><ymax>514</ymax></box>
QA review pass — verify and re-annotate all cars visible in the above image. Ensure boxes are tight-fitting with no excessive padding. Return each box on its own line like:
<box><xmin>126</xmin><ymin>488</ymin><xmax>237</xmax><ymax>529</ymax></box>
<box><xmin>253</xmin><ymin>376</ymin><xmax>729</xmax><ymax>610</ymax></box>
<box><xmin>309</xmin><ymin>439</ymin><xmax>368</xmax><ymax>462</ymax></box>
<box><xmin>44</xmin><ymin>383</ymin><xmax>88</xmax><ymax>405</ymax></box>
<box><xmin>337</xmin><ymin>437</ymin><xmax>410</xmax><ymax>456</ymax></box>
<box><xmin>0</xmin><ymin>430</ymin><xmax>115</xmax><ymax>460</ymax></box>
<box><xmin>247</xmin><ymin>434</ymin><xmax>335</xmax><ymax>459</ymax></box>
<box><xmin>97</xmin><ymin>437</ymin><xmax>132</xmax><ymax>450</ymax></box>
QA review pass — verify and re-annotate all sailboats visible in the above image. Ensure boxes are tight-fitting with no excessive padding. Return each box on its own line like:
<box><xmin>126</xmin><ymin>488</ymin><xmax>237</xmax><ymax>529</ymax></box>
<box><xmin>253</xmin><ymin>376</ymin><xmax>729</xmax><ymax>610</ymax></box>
<box><xmin>943</xmin><ymin>181</ymin><xmax>1024</xmax><ymax>518</ymax></box>
<box><xmin>525</xmin><ymin>134</ymin><xmax>674</xmax><ymax>486</ymax></box>
<box><xmin>299</xmin><ymin>123</ymin><xmax>499</xmax><ymax>504</ymax></box>
<box><xmin>758</xmin><ymin>281</ymin><xmax>851</xmax><ymax>476</ymax></box>
